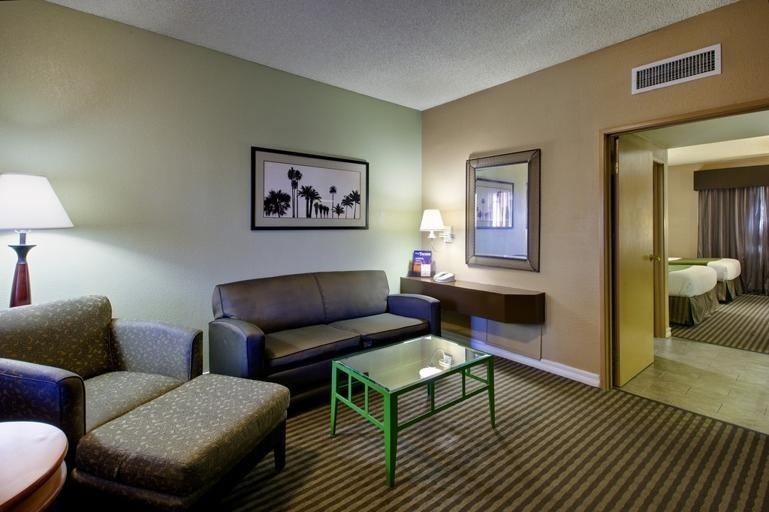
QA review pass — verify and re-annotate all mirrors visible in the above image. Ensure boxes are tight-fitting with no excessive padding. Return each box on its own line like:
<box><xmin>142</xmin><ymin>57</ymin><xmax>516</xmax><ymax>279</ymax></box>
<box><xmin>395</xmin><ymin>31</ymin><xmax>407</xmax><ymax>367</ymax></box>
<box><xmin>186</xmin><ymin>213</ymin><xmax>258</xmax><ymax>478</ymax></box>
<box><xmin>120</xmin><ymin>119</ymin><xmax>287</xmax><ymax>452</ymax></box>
<box><xmin>465</xmin><ymin>148</ymin><xmax>542</xmax><ymax>273</ymax></box>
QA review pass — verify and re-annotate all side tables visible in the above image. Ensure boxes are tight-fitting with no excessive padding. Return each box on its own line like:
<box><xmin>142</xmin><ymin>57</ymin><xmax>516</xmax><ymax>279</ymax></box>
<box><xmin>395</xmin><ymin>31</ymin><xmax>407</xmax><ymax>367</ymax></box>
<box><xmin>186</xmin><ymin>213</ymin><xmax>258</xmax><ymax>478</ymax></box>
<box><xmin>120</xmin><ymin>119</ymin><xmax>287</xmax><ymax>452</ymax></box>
<box><xmin>0</xmin><ymin>420</ymin><xmax>68</xmax><ymax>512</ymax></box>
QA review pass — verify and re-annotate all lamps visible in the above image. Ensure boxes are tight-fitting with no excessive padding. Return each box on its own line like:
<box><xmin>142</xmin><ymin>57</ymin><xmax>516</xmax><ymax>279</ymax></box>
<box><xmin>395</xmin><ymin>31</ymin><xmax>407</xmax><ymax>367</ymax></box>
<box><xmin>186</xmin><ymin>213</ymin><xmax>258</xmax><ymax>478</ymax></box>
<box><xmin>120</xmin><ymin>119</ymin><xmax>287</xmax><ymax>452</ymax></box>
<box><xmin>418</xmin><ymin>209</ymin><xmax>446</xmax><ymax>239</ymax></box>
<box><xmin>0</xmin><ymin>173</ymin><xmax>73</xmax><ymax>308</ymax></box>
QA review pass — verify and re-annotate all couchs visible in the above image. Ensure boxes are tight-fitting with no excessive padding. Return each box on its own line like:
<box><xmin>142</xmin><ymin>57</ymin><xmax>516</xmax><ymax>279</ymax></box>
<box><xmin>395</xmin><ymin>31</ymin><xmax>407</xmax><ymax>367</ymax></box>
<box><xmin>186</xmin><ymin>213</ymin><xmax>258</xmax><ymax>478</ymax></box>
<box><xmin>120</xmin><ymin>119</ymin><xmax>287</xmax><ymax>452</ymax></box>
<box><xmin>0</xmin><ymin>293</ymin><xmax>203</xmax><ymax>456</ymax></box>
<box><xmin>208</xmin><ymin>268</ymin><xmax>442</xmax><ymax>413</ymax></box>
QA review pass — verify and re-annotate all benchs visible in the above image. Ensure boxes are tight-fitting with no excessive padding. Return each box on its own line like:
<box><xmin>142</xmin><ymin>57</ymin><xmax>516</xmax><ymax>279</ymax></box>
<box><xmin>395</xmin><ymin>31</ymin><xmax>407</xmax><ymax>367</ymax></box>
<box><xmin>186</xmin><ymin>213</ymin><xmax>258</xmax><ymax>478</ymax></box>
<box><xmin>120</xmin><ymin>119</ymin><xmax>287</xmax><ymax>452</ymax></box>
<box><xmin>74</xmin><ymin>374</ymin><xmax>291</xmax><ymax>512</ymax></box>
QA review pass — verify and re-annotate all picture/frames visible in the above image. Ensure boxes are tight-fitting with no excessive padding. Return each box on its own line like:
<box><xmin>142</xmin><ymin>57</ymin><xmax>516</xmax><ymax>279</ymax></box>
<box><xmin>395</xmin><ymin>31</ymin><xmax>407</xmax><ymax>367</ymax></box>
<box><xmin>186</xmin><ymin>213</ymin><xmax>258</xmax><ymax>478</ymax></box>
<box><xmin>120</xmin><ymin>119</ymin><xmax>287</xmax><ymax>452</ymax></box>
<box><xmin>476</xmin><ymin>177</ymin><xmax>514</xmax><ymax>229</ymax></box>
<box><xmin>250</xmin><ymin>146</ymin><xmax>369</xmax><ymax>231</ymax></box>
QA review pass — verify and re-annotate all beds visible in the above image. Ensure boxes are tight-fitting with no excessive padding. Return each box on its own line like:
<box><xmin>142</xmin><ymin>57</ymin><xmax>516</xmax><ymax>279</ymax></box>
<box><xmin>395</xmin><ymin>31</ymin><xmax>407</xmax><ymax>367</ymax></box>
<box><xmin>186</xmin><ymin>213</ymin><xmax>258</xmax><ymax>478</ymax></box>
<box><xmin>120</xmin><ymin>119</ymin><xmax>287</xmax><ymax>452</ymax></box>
<box><xmin>668</xmin><ymin>264</ymin><xmax>720</xmax><ymax>329</ymax></box>
<box><xmin>668</xmin><ymin>257</ymin><xmax>741</xmax><ymax>302</ymax></box>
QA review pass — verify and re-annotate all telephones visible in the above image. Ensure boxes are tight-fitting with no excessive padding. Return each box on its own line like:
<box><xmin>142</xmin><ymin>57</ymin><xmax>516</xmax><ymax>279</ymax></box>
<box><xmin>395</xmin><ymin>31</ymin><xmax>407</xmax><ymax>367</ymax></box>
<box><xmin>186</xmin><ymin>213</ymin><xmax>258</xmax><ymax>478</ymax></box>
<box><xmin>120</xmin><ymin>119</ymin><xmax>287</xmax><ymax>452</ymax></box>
<box><xmin>433</xmin><ymin>272</ymin><xmax>455</xmax><ymax>282</ymax></box>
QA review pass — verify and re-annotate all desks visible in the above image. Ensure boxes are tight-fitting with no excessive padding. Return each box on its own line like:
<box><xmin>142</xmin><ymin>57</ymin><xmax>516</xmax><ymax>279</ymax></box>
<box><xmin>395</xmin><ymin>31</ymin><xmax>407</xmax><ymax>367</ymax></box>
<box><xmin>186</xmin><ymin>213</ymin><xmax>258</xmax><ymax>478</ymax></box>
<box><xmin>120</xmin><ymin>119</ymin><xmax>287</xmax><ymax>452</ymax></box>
<box><xmin>400</xmin><ymin>276</ymin><xmax>545</xmax><ymax>362</ymax></box>
